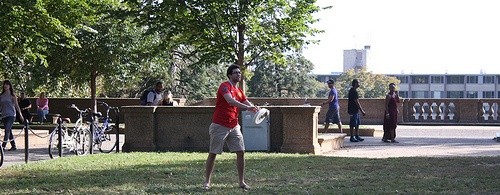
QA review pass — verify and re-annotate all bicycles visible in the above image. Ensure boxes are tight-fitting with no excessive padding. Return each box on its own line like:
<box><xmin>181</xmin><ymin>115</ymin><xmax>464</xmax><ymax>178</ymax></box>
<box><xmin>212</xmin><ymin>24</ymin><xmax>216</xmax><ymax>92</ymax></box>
<box><xmin>0</xmin><ymin>143</ymin><xmax>3</xmax><ymax>167</ymax></box>
<box><xmin>85</xmin><ymin>101</ymin><xmax>121</xmax><ymax>153</ymax></box>
<box><xmin>48</xmin><ymin>104</ymin><xmax>92</xmax><ymax>159</ymax></box>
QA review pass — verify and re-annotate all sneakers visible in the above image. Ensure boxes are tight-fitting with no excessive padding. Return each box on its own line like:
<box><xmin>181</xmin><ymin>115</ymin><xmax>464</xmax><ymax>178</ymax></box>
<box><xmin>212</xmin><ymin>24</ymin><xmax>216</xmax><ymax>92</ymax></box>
<box><xmin>355</xmin><ymin>137</ymin><xmax>364</xmax><ymax>141</ymax></box>
<box><xmin>350</xmin><ymin>136</ymin><xmax>358</xmax><ymax>142</ymax></box>
<box><xmin>8</xmin><ymin>148</ymin><xmax>16</xmax><ymax>151</ymax></box>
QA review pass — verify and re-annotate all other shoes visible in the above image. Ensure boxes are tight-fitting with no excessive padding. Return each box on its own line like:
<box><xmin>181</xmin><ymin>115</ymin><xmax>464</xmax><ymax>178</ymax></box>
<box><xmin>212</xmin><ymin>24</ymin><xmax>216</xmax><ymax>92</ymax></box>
<box><xmin>391</xmin><ymin>140</ymin><xmax>399</xmax><ymax>143</ymax></box>
<box><xmin>382</xmin><ymin>139</ymin><xmax>390</xmax><ymax>143</ymax></box>
<box><xmin>239</xmin><ymin>183</ymin><xmax>250</xmax><ymax>188</ymax></box>
<box><xmin>203</xmin><ymin>183</ymin><xmax>209</xmax><ymax>191</ymax></box>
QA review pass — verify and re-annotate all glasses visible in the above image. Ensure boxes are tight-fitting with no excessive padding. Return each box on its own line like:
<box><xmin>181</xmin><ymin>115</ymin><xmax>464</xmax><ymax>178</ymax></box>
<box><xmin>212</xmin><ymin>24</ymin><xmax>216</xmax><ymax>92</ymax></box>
<box><xmin>328</xmin><ymin>83</ymin><xmax>333</xmax><ymax>84</ymax></box>
<box><xmin>231</xmin><ymin>72</ymin><xmax>242</xmax><ymax>74</ymax></box>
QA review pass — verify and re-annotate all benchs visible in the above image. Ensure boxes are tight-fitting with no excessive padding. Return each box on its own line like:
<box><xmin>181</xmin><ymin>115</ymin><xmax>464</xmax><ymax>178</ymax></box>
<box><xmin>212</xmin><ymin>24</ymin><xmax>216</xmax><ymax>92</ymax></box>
<box><xmin>29</xmin><ymin>113</ymin><xmax>61</xmax><ymax>124</ymax></box>
